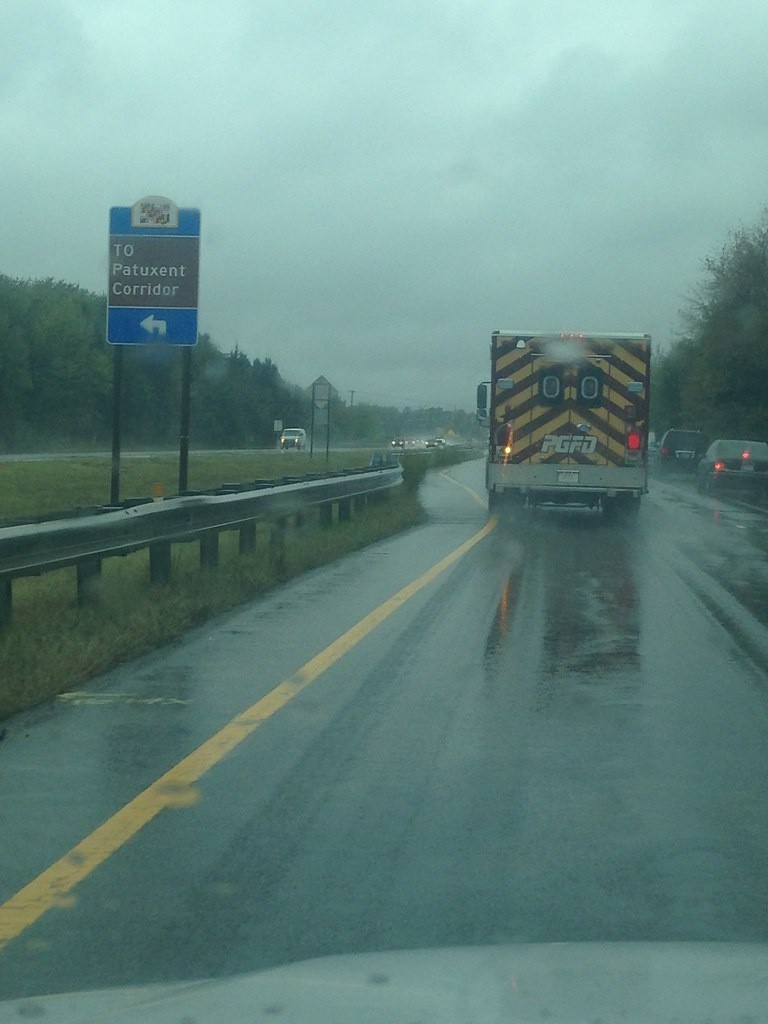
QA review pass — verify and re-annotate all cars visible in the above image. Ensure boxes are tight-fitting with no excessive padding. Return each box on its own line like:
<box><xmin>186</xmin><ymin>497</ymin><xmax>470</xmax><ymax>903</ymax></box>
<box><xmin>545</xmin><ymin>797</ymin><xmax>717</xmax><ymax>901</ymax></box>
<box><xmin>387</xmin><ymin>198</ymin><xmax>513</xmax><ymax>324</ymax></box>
<box><xmin>696</xmin><ymin>439</ymin><xmax>768</xmax><ymax>499</ymax></box>
<box><xmin>391</xmin><ymin>437</ymin><xmax>450</xmax><ymax>449</ymax></box>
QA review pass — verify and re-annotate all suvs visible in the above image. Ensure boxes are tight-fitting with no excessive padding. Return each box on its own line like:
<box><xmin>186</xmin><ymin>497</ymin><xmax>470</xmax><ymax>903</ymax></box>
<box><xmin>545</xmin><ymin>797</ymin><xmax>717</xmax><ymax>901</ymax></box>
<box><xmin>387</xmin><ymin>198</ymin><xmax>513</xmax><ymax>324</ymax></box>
<box><xmin>657</xmin><ymin>430</ymin><xmax>710</xmax><ymax>474</ymax></box>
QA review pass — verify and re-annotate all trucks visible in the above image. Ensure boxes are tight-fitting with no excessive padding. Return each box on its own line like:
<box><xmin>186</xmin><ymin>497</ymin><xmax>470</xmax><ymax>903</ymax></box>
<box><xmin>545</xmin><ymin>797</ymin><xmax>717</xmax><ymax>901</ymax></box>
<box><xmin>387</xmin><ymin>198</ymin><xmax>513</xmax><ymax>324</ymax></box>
<box><xmin>476</xmin><ymin>328</ymin><xmax>651</xmax><ymax>514</ymax></box>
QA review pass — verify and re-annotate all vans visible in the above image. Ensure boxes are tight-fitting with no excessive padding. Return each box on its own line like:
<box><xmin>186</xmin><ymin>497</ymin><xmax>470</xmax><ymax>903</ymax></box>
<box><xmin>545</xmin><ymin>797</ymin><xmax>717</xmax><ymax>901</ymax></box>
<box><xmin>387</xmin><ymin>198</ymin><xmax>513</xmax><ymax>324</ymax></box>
<box><xmin>281</xmin><ymin>429</ymin><xmax>305</xmax><ymax>450</ymax></box>
